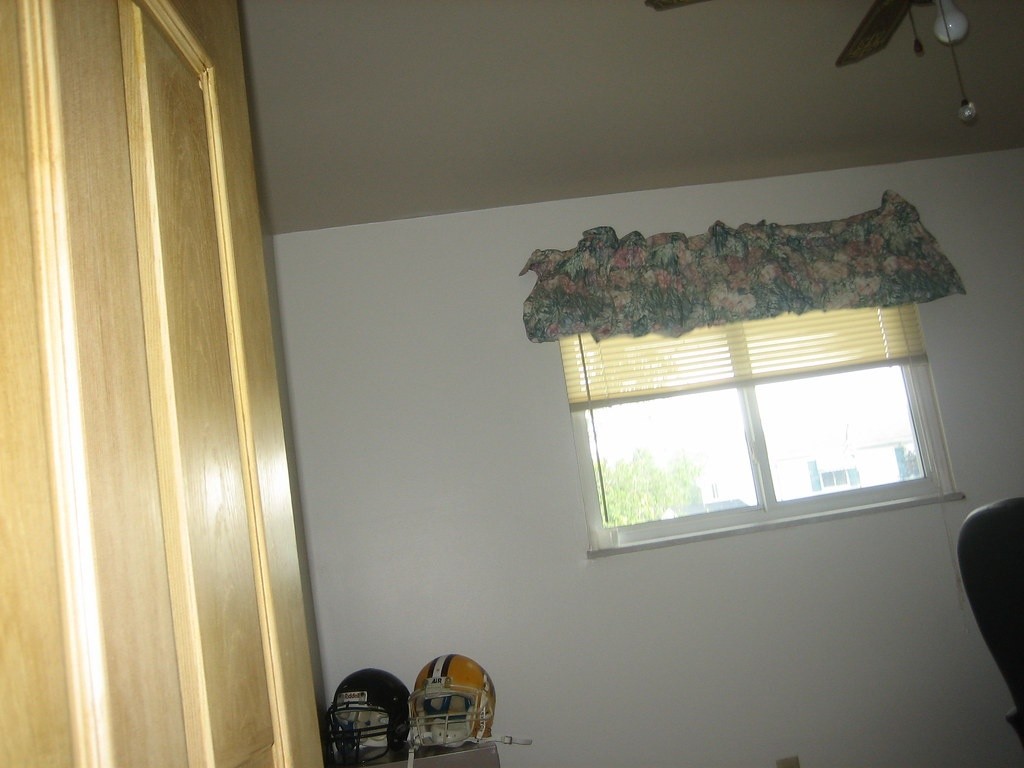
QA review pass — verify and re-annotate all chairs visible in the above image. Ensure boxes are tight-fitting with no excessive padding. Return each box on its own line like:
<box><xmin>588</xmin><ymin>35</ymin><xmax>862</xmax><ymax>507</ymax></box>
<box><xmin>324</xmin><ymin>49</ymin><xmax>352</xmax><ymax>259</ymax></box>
<box><xmin>957</xmin><ymin>496</ymin><xmax>1024</xmax><ymax>743</ymax></box>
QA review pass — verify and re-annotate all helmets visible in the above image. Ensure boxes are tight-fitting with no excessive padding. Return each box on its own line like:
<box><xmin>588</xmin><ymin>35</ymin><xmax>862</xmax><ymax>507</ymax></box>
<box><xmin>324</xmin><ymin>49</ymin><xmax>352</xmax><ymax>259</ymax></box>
<box><xmin>407</xmin><ymin>654</ymin><xmax>497</xmax><ymax>749</ymax></box>
<box><xmin>321</xmin><ymin>668</ymin><xmax>413</xmax><ymax>766</ymax></box>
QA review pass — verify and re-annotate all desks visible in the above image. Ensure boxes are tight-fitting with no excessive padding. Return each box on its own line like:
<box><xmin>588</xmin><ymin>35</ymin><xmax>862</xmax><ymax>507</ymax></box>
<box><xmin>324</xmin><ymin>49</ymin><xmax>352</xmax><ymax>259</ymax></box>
<box><xmin>332</xmin><ymin>736</ymin><xmax>501</xmax><ymax>768</ymax></box>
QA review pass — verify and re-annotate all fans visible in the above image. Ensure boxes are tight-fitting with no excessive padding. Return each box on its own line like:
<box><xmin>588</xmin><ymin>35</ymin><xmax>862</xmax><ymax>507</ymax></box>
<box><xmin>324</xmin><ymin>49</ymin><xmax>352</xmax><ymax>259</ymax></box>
<box><xmin>643</xmin><ymin>0</ymin><xmax>934</xmax><ymax>68</ymax></box>
